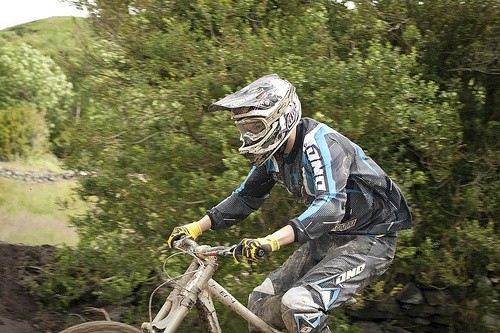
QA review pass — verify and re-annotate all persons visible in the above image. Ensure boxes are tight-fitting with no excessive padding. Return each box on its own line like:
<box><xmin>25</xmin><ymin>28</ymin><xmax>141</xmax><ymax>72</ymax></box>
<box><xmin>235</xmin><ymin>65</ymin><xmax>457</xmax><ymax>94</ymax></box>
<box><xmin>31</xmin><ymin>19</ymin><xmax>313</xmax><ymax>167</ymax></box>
<box><xmin>167</xmin><ymin>73</ymin><xmax>412</xmax><ymax>332</ymax></box>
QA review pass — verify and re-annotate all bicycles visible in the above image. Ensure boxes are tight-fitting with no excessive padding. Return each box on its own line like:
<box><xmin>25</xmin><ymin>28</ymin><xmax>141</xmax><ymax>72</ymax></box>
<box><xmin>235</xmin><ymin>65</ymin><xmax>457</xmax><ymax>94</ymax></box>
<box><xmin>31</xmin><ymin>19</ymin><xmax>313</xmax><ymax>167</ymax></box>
<box><xmin>56</xmin><ymin>236</ymin><xmax>287</xmax><ymax>333</ymax></box>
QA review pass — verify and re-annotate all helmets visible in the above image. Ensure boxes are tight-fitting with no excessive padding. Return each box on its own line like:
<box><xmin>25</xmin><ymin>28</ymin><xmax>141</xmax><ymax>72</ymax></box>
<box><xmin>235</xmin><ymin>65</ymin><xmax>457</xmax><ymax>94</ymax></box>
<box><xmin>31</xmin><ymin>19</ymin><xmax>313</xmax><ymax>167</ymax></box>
<box><xmin>208</xmin><ymin>74</ymin><xmax>301</xmax><ymax>168</ymax></box>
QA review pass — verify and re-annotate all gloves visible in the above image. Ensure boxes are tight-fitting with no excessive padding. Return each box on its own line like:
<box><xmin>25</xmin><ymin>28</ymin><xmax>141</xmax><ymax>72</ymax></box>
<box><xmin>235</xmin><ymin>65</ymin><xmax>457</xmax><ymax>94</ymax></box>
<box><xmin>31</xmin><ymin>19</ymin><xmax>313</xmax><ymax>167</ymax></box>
<box><xmin>168</xmin><ymin>222</ymin><xmax>202</xmax><ymax>250</ymax></box>
<box><xmin>233</xmin><ymin>235</ymin><xmax>280</xmax><ymax>268</ymax></box>
